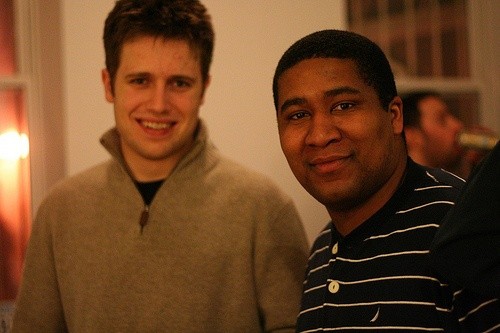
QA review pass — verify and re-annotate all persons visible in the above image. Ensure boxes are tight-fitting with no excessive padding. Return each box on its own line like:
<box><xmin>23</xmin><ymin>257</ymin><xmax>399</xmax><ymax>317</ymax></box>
<box><xmin>273</xmin><ymin>30</ymin><xmax>500</xmax><ymax>333</ymax></box>
<box><xmin>400</xmin><ymin>91</ymin><xmax>492</xmax><ymax>172</ymax></box>
<box><xmin>10</xmin><ymin>0</ymin><xmax>310</xmax><ymax>333</ymax></box>
